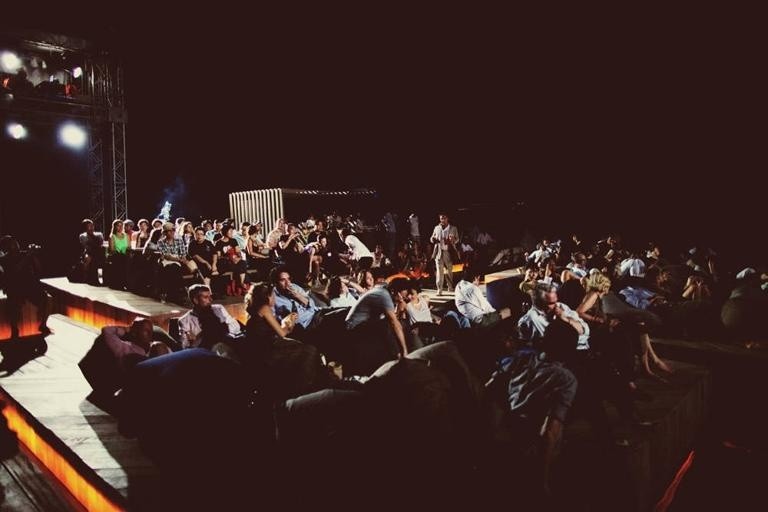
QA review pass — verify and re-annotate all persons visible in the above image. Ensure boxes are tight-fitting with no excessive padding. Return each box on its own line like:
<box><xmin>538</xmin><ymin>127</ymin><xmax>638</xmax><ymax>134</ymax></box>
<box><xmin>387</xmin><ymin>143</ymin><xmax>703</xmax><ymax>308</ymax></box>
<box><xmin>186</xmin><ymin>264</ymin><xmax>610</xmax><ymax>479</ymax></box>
<box><xmin>0</xmin><ymin>235</ymin><xmax>55</xmax><ymax>345</ymax></box>
